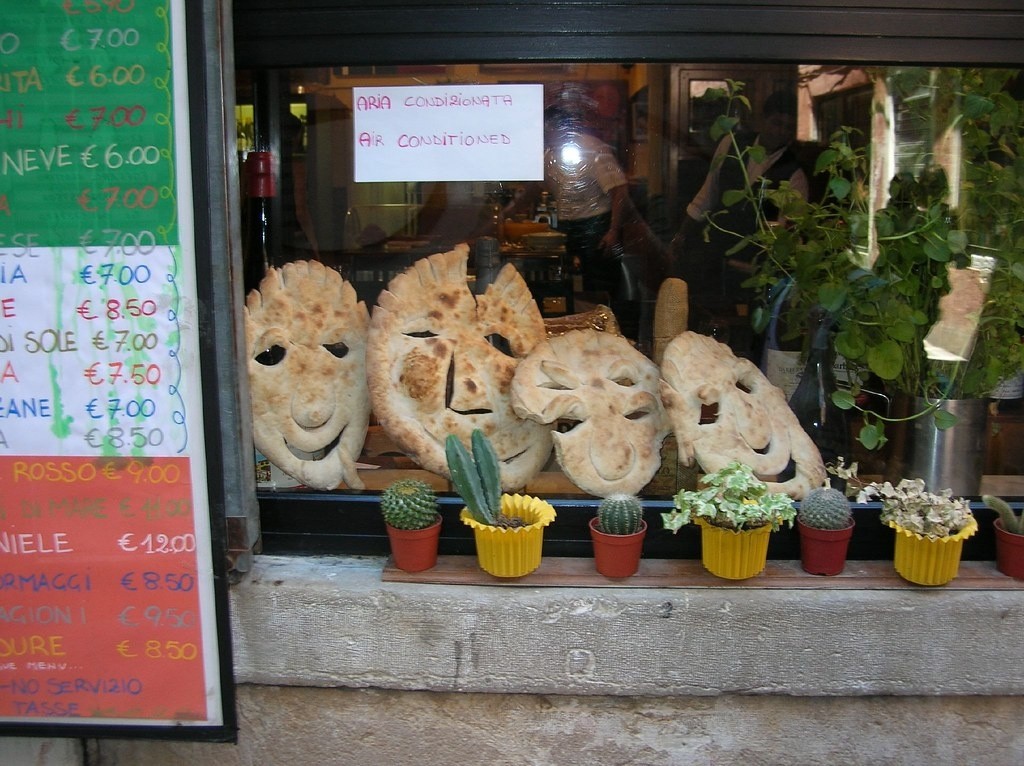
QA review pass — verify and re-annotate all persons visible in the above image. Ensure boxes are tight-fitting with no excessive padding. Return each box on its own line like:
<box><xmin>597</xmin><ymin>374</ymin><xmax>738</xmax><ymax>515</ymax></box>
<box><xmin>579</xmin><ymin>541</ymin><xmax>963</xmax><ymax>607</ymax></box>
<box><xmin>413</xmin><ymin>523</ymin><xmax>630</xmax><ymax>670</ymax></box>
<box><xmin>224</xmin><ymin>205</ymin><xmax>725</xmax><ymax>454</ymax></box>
<box><xmin>473</xmin><ymin>102</ymin><xmax>641</xmax><ymax>343</ymax></box>
<box><xmin>673</xmin><ymin>90</ymin><xmax>810</xmax><ymax>364</ymax></box>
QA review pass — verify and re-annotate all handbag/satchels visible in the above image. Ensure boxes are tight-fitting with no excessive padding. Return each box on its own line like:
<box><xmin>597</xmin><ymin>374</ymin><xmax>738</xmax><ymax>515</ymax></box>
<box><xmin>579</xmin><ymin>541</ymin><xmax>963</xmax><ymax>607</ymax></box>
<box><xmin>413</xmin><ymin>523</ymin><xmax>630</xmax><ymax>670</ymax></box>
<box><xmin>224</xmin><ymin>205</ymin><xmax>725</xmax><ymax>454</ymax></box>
<box><xmin>767</xmin><ymin>349</ymin><xmax>863</xmax><ymax>404</ymax></box>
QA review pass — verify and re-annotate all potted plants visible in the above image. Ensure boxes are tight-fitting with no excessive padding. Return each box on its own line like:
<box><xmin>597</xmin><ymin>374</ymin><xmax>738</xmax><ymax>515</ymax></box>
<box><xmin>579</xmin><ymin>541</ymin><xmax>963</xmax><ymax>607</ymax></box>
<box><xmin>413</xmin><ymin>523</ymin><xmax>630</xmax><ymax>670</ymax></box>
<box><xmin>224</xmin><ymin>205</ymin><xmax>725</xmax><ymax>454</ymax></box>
<box><xmin>444</xmin><ymin>428</ymin><xmax>557</xmax><ymax>578</ymax></box>
<box><xmin>698</xmin><ymin>63</ymin><xmax>1024</xmax><ymax>499</ymax></box>
<box><xmin>588</xmin><ymin>492</ymin><xmax>647</xmax><ymax>577</ymax></box>
<box><xmin>660</xmin><ymin>460</ymin><xmax>797</xmax><ymax>580</ymax></box>
<box><xmin>796</xmin><ymin>487</ymin><xmax>856</xmax><ymax>575</ymax></box>
<box><xmin>822</xmin><ymin>456</ymin><xmax>979</xmax><ymax>586</ymax></box>
<box><xmin>380</xmin><ymin>475</ymin><xmax>444</xmax><ymax>573</ymax></box>
<box><xmin>981</xmin><ymin>494</ymin><xmax>1024</xmax><ymax>579</ymax></box>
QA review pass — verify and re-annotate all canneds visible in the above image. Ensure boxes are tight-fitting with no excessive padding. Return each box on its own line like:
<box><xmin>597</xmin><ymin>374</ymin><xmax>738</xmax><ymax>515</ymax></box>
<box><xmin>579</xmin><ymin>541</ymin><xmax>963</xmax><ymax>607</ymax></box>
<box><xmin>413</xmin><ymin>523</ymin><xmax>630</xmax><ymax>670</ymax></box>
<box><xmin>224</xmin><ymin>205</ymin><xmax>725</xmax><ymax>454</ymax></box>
<box><xmin>475</xmin><ymin>236</ymin><xmax>497</xmax><ymax>268</ymax></box>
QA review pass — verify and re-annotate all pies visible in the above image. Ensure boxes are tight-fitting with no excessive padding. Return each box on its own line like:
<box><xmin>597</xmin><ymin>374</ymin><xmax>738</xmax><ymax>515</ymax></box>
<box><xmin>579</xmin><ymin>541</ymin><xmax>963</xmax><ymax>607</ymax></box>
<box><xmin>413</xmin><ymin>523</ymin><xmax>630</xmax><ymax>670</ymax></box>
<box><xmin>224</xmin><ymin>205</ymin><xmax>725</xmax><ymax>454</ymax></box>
<box><xmin>366</xmin><ymin>243</ymin><xmax>558</xmax><ymax>492</ymax></box>
<box><xmin>244</xmin><ymin>258</ymin><xmax>377</xmax><ymax>490</ymax></box>
<box><xmin>511</xmin><ymin>329</ymin><xmax>676</xmax><ymax>498</ymax></box>
<box><xmin>659</xmin><ymin>330</ymin><xmax>827</xmax><ymax>500</ymax></box>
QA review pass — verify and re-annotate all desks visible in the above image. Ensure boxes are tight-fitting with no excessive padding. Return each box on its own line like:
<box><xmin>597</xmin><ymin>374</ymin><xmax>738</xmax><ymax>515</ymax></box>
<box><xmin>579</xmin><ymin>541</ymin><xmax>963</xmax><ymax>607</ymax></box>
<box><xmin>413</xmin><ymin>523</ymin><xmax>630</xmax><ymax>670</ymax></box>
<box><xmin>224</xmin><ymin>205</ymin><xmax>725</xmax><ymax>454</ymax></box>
<box><xmin>347</xmin><ymin>230</ymin><xmax>575</xmax><ymax>320</ymax></box>
